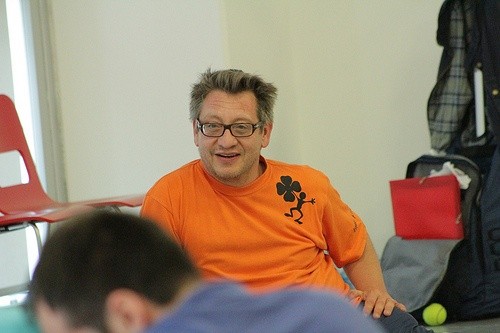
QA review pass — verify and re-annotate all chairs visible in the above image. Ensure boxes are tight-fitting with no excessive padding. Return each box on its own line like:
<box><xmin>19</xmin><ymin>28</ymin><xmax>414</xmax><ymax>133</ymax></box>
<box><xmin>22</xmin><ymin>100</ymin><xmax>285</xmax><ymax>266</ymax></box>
<box><xmin>0</xmin><ymin>93</ymin><xmax>147</xmax><ymax>260</ymax></box>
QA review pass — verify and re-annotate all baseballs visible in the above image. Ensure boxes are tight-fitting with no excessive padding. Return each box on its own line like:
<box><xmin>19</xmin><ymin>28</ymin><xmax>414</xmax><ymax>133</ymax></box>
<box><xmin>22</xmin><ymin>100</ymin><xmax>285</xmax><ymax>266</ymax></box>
<box><xmin>424</xmin><ymin>303</ymin><xmax>447</xmax><ymax>326</ymax></box>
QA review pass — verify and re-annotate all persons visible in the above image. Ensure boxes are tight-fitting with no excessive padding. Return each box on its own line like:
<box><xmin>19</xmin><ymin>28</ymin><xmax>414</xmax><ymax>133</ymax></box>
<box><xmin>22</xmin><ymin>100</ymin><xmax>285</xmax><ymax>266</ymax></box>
<box><xmin>140</xmin><ymin>66</ymin><xmax>434</xmax><ymax>333</ymax></box>
<box><xmin>0</xmin><ymin>211</ymin><xmax>388</xmax><ymax>333</ymax></box>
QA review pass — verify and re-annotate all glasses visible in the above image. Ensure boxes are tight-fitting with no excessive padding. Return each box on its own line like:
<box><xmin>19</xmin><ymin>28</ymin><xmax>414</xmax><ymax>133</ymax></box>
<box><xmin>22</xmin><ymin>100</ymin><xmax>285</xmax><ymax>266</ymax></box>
<box><xmin>195</xmin><ymin>120</ymin><xmax>261</xmax><ymax>138</ymax></box>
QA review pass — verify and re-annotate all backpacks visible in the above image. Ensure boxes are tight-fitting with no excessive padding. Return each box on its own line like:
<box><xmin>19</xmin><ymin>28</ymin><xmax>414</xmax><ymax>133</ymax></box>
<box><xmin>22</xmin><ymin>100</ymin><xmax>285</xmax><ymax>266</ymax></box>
<box><xmin>380</xmin><ymin>153</ymin><xmax>500</xmax><ymax>323</ymax></box>
